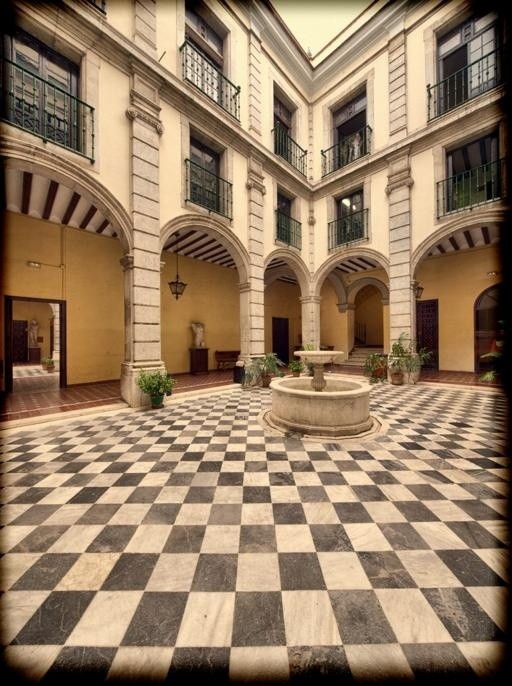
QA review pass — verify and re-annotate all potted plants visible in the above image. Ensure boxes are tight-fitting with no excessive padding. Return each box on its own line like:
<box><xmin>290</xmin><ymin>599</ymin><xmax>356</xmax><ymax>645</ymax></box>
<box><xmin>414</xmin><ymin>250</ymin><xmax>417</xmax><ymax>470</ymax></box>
<box><xmin>137</xmin><ymin>368</ymin><xmax>175</xmax><ymax>409</ymax></box>
<box><xmin>288</xmin><ymin>360</ymin><xmax>305</xmax><ymax>377</ymax></box>
<box><xmin>388</xmin><ymin>332</ymin><xmax>433</xmax><ymax>385</ymax></box>
<box><xmin>364</xmin><ymin>354</ymin><xmax>387</xmax><ymax>384</ymax></box>
<box><xmin>40</xmin><ymin>357</ymin><xmax>48</xmax><ymax>370</ymax></box>
<box><xmin>240</xmin><ymin>353</ymin><xmax>286</xmax><ymax>388</ymax></box>
<box><xmin>45</xmin><ymin>359</ymin><xmax>56</xmax><ymax>373</ymax></box>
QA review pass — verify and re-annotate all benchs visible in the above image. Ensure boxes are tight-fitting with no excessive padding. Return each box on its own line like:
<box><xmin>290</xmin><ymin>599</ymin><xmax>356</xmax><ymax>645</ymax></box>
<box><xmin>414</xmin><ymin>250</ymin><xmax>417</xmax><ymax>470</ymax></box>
<box><xmin>215</xmin><ymin>350</ymin><xmax>240</xmax><ymax>369</ymax></box>
<box><xmin>215</xmin><ymin>350</ymin><xmax>240</xmax><ymax>372</ymax></box>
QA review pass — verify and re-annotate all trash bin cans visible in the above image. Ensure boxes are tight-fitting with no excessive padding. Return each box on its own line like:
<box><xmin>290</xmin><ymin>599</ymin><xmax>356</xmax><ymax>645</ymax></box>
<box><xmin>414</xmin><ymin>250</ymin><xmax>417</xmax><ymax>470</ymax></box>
<box><xmin>233</xmin><ymin>361</ymin><xmax>245</xmax><ymax>382</ymax></box>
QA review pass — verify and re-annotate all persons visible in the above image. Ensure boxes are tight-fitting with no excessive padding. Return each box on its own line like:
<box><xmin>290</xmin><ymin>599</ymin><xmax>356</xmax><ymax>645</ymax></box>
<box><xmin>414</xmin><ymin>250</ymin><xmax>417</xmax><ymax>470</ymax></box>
<box><xmin>24</xmin><ymin>318</ymin><xmax>39</xmax><ymax>347</ymax></box>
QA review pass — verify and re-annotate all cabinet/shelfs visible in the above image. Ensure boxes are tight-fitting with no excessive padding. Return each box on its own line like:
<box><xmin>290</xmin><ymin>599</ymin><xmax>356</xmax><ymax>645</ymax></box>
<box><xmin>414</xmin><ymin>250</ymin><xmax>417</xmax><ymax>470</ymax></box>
<box><xmin>189</xmin><ymin>348</ymin><xmax>209</xmax><ymax>375</ymax></box>
<box><xmin>29</xmin><ymin>348</ymin><xmax>41</xmax><ymax>363</ymax></box>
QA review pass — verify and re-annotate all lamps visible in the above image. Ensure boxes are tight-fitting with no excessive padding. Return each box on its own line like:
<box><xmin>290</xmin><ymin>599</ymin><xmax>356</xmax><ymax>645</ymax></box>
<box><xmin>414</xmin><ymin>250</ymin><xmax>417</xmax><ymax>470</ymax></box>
<box><xmin>168</xmin><ymin>233</ymin><xmax>188</xmax><ymax>300</ymax></box>
<box><xmin>415</xmin><ymin>286</ymin><xmax>424</xmax><ymax>298</ymax></box>
